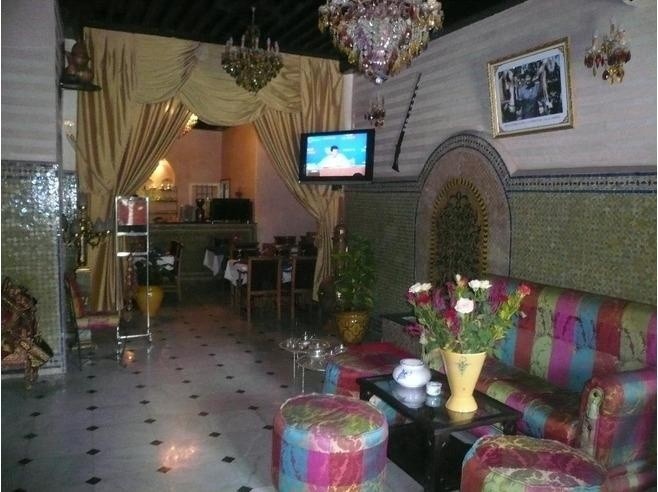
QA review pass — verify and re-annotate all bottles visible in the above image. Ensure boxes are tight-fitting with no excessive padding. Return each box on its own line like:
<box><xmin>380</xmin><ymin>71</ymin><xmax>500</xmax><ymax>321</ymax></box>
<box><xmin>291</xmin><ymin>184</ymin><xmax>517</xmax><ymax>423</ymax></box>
<box><xmin>194</xmin><ymin>197</ymin><xmax>205</xmax><ymax>221</ymax></box>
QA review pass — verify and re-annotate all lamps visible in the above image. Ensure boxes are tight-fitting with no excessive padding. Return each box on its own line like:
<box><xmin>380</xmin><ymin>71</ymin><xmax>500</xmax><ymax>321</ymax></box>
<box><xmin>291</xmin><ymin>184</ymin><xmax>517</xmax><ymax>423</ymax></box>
<box><xmin>363</xmin><ymin>103</ymin><xmax>385</xmax><ymax>128</ymax></box>
<box><xmin>220</xmin><ymin>0</ymin><xmax>445</xmax><ymax>96</ymax></box>
<box><xmin>582</xmin><ymin>16</ymin><xmax>632</xmax><ymax>87</ymax></box>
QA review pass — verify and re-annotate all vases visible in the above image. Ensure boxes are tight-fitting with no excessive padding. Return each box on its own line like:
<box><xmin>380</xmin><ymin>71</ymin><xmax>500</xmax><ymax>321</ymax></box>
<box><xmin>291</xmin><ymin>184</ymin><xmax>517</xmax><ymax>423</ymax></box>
<box><xmin>439</xmin><ymin>347</ymin><xmax>487</xmax><ymax>413</ymax></box>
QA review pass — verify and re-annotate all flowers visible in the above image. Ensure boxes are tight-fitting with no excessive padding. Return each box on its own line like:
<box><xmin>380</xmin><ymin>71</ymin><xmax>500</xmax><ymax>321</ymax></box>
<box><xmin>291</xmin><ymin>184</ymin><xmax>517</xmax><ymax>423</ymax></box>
<box><xmin>401</xmin><ymin>272</ymin><xmax>531</xmax><ymax>358</ymax></box>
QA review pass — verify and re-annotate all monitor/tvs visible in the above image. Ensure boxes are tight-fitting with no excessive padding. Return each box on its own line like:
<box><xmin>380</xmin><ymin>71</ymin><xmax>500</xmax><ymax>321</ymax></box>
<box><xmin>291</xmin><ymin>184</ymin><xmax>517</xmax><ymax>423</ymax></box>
<box><xmin>299</xmin><ymin>128</ymin><xmax>376</xmax><ymax>181</ymax></box>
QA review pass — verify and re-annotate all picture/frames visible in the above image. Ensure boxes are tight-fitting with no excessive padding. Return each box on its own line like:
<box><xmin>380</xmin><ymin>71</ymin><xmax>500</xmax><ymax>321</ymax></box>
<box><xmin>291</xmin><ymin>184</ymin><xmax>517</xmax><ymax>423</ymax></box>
<box><xmin>487</xmin><ymin>37</ymin><xmax>575</xmax><ymax>140</ymax></box>
<box><xmin>188</xmin><ymin>178</ymin><xmax>231</xmax><ymax>223</ymax></box>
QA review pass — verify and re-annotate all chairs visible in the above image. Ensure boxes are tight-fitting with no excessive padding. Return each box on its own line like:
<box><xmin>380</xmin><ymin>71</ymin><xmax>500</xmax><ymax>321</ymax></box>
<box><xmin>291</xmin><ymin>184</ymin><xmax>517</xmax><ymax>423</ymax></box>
<box><xmin>148</xmin><ymin>240</ymin><xmax>184</xmax><ymax>303</ymax></box>
<box><xmin>64</xmin><ymin>274</ymin><xmax>128</xmax><ymax>372</ymax></box>
<box><xmin>230</xmin><ymin>235</ymin><xmax>323</xmax><ymax>323</ymax></box>
<box><xmin>1</xmin><ymin>275</ymin><xmax>39</xmax><ymax>391</ymax></box>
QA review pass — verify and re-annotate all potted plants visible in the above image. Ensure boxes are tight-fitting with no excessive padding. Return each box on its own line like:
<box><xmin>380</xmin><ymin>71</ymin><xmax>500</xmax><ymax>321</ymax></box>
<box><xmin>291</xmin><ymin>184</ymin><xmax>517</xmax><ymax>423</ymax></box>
<box><xmin>325</xmin><ymin>232</ymin><xmax>377</xmax><ymax>346</ymax></box>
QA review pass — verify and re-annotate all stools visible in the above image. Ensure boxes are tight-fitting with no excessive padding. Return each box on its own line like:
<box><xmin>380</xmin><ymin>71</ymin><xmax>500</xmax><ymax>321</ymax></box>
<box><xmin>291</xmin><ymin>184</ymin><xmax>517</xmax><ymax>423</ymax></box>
<box><xmin>270</xmin><ymin>351</ymin><xmax>407</xmax><ymax>492</ymax></box>
<box><xmin>460</xmin><ymin>433</ymin><xmax>606</xmax><ymax>492</ymax></box>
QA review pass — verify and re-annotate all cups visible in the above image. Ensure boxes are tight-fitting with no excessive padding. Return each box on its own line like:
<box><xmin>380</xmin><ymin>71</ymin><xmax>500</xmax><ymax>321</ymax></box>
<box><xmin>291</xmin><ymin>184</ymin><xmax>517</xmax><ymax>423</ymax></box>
<box><xmin>424</xmin><ymin>380</ymin><xmax>443</xmax><ymax>398</ymax></box>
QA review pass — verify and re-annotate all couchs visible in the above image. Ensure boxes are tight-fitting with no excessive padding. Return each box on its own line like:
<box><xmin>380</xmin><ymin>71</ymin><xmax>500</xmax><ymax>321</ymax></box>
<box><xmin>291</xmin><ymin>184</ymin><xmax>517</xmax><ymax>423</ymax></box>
<box><xmin>425</xmin><ymin>271</ymin><xmax>657</xmax><ymax>492</ymax></box>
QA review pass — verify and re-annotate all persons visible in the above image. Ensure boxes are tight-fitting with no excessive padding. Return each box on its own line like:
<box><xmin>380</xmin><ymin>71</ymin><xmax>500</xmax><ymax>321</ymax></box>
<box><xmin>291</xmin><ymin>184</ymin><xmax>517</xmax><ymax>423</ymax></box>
<box><xmin>517</xmin><ymin>69</ymin><xmax>545</xmax><ymax>120</ymax></box>
<box><xmin>315</xmin><ymin>147</ymin><xmax>354</xmax><ymax>167</ymax></box>
